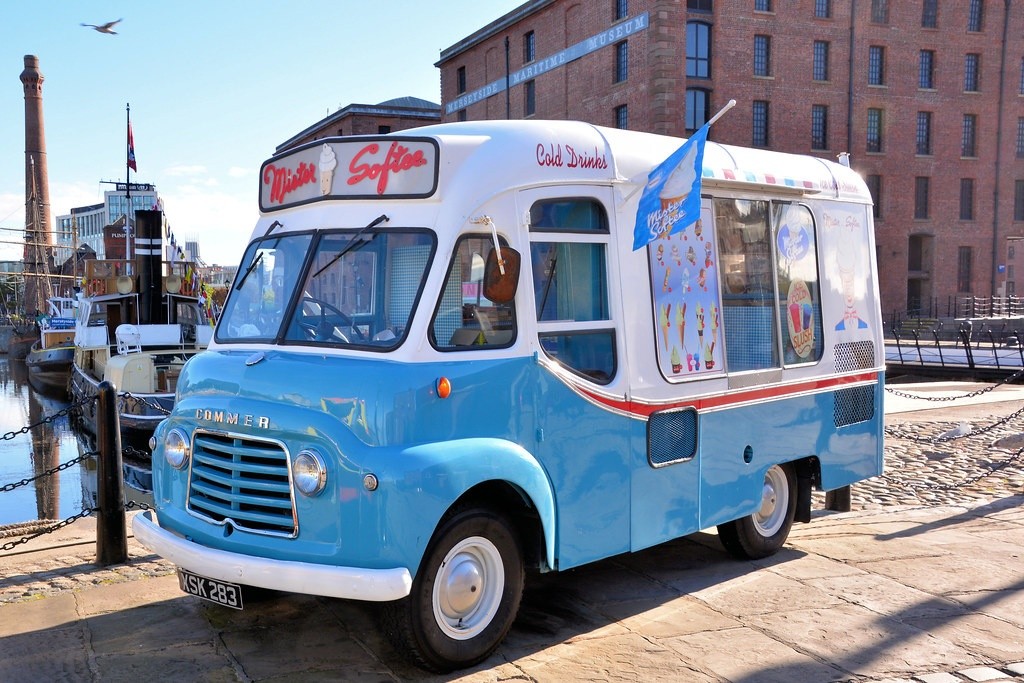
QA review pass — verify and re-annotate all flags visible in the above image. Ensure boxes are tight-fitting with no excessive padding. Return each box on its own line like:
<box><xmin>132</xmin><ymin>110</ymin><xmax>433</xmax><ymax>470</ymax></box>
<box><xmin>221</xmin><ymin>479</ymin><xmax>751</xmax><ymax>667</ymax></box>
<box><xmin>156</xmin><ymin>197</ymin><xmax>218</xmax><ymax>328</ymax></box>
<box><xmin>128</xmin><ymin>122</ymin><xmax>137</xmax><ymax>173</ymax></box>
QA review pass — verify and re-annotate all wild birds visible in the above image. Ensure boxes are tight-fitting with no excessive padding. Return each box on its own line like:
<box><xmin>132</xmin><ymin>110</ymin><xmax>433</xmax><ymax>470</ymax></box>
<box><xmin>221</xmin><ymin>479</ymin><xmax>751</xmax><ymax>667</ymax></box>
<box><xmin>80</xmin><ymin>18</ymin><xmax>122</xmax><ymax>35</ymax></box>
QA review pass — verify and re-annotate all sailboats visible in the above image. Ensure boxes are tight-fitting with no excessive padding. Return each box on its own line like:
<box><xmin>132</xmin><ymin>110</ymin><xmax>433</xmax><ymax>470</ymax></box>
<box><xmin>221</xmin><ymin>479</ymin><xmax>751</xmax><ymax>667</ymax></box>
<box><xmin>1</xmin><ymin>154</ymin><xmax>85</xmax><ymax>361</ymax></box>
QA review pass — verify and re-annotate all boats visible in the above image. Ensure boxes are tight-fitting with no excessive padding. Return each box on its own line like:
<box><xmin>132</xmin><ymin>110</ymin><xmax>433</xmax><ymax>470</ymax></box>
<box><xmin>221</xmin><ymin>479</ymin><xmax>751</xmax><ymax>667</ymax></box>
<box><xmin>68</xmin><ymin>103</ymin><xmax>216</xmax><ymax>439</ymax></box>
<box><xmin>25</xmin><ymin>297</ymin><xmax>79</xmax><ymax>402</ymax></box>
<box><xmin>73</xmin><ymin>430</ymin><xmax>155</xmax><ymax>517</ymax></box>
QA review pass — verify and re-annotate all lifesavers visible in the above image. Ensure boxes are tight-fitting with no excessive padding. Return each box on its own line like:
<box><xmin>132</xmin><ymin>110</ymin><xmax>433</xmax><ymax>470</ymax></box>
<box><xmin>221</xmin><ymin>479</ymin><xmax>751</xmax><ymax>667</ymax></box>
<box><xmin>180</xmin><ymin>280</ymin><xmax>193</xmax><ymax>295</ymax></box>
<box><xmin>88</xmin><ymin>279</ymin><xmax>105</xmax><ymax>296</ymax></box>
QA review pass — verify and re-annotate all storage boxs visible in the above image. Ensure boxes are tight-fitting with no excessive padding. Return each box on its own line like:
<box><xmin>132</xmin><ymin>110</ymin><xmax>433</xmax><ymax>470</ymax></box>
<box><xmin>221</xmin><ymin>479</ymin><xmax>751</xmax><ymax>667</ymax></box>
<box><xmin>448</xmin><ymin>327</ymin><xmax>515</xmax><ymax>348</ymax></box>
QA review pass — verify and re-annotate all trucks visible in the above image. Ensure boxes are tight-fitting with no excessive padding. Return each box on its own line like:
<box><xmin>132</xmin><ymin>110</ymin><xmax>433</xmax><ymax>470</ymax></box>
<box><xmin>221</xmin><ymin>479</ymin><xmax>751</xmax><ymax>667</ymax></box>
<box><xmin>132</xmin><ymin>118</ymin><xmax>886</xmax><ymax>675</ymax></box>
